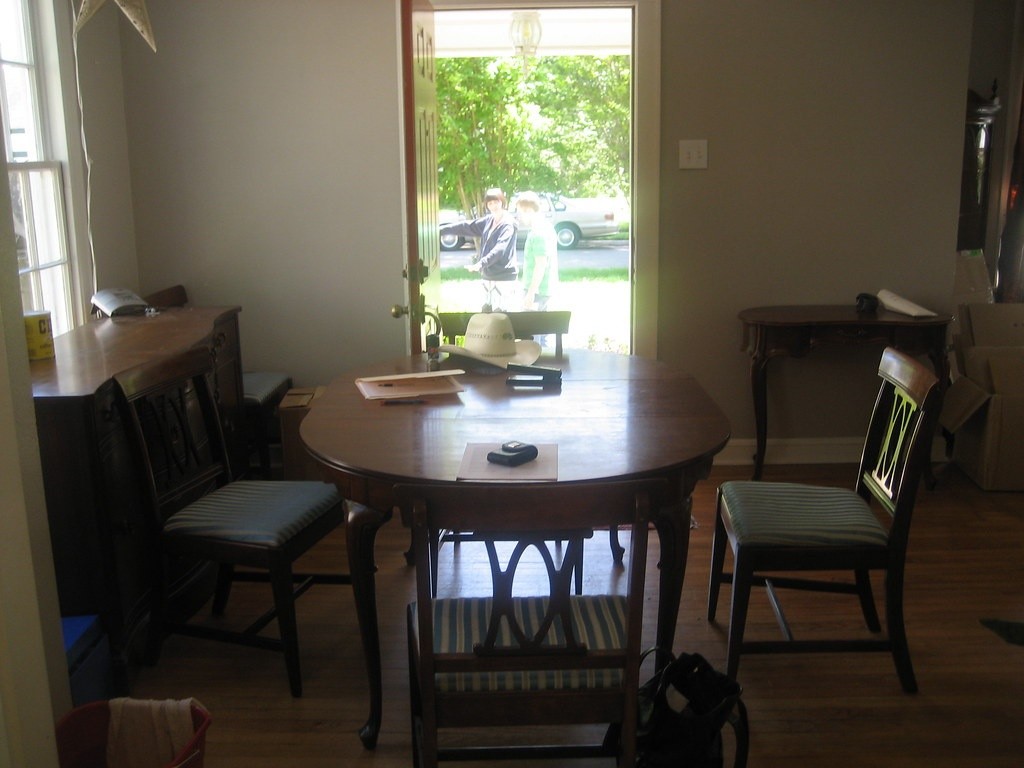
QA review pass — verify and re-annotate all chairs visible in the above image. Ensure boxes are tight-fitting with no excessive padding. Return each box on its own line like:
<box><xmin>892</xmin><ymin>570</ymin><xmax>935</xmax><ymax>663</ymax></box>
<box><xmin>67</xmin><ymin>359</ymin><xmax>354</xmax><ymax>696</ymax></box>
<box><xmin>111</xmin><ymin>285</ymin><xmax>295</xmax><ymax>473</ymax></box>
<box><xmin>705</xmin><ymin>352</ymin><xmax>940</xmax><ymax>694</ymax></box>
<box><xmin>430</xmin><ymin>309</ymin><xmax>595</xmax><ymax>597</ymax></box>
<box><xmin>381</xmin><ymin>468</ymin><xmax>675</xmax><ymax>764</ymax></box>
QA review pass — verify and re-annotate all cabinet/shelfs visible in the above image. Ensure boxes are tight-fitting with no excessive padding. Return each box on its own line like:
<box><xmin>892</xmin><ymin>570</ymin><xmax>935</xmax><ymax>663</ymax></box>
<box><xmin>29</xmin><ymin>302</ymin><xmax>248</xmax><ymax>635</ymax></box>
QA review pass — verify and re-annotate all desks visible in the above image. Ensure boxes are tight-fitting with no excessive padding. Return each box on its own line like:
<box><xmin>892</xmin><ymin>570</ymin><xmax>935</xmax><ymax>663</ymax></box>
<box><xmin>300</xmin><ymin>353</ymin><xmax>730</xmax><ymax>768</ymax></box>
<box><xmin>737</xmin><ymin>307</ymin><xmax>957</xmax><ymax>496</ymax></box>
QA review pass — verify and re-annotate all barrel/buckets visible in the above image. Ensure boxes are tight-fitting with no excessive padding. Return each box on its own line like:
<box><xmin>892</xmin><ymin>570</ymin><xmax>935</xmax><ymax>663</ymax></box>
<box><xmin>24</xmin><ymin>311</ymin><xmax>55</xmax><ymax>360</ymax></box>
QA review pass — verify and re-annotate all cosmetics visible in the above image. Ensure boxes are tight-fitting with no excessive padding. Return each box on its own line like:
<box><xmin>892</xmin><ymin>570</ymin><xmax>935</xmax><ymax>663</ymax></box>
<box><xmin>850</xmin><ymin>290</ymin><xmax>939</xmax><ymax>325</ymax></box>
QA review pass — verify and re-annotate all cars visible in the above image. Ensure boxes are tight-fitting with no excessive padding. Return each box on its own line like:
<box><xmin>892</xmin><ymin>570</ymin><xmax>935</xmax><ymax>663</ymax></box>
<box><xmin>439</xmin><ymin>191</ymin><xmax>622</xmax><ymax>253</ymax></box>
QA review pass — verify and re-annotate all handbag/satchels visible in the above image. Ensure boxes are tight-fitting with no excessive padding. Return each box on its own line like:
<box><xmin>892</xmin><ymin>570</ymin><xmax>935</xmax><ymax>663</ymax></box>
<box><xmin>602</xmin><ymin>646</ymin><xmax>749</xmax><ymax>768</ymax></box>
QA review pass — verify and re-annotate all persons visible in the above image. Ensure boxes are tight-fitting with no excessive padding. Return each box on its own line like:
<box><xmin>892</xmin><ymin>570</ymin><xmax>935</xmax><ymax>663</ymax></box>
<box><xmin>440</xmin><ymin>187</ymin><xmax>517</xmax><ymax>310</ymax></box>
<box><xmin>509</xmin><ymin>191</ymin><xmax>559</xmax><ymax>350</ymax></box>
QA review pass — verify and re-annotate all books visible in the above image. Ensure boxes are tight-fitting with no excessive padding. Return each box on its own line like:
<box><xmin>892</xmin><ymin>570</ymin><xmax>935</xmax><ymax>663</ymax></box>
<box><xmin>354</xmin><ymin>369</ymin><xmax>465</xmax><ymax>401</ymax></box>
<box><xmin>456</xmin><ymin>441</ymin><xmax>558</xmax><ymax>484</ymax></box>
<box><xmin>92</xmin><ymin>286</ymin><xmax>147</xmax><ymax>317</ymax></box>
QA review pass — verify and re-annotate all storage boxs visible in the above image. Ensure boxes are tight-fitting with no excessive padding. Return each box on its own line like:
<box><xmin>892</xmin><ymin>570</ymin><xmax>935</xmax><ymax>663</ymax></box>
<box><xmin>935</xmin><ymin>302</ymin><xmax>1023</xmax><ymax>492</ymax></box>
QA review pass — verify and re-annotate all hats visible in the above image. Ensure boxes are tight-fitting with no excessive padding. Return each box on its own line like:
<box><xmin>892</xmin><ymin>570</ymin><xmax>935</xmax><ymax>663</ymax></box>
<box><xmin>437</xmin><ymin>313</ymin><xmax>542</xmax><ymax>369</ymax></box>
